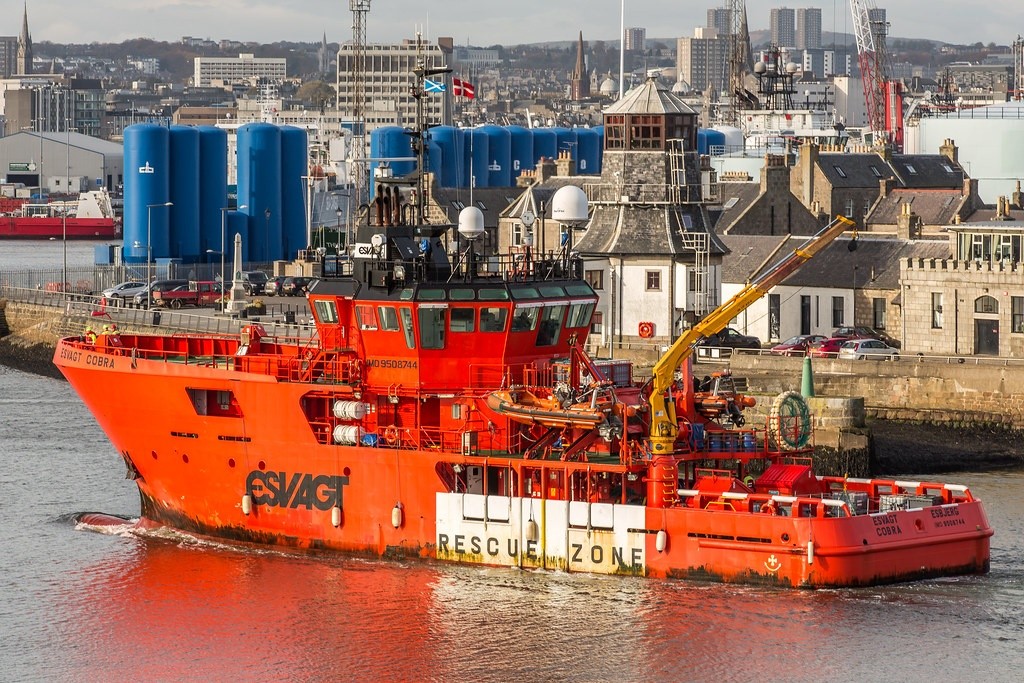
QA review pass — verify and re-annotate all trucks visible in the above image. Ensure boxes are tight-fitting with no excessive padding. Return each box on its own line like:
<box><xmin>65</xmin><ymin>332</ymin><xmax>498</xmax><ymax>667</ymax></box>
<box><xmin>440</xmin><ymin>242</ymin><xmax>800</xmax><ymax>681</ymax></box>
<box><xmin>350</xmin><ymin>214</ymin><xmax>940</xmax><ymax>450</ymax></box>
<box><xmin>154</xmin><ymin>281</ymin><xmax>230</xmax><ymax>308</ymax></box>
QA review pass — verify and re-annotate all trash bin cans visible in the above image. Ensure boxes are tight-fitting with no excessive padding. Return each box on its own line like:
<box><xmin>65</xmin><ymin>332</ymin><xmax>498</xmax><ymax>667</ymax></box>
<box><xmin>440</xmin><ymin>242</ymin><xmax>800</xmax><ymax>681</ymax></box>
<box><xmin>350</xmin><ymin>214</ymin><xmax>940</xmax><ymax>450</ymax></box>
<box><xmin>283</xmin><ymin>310</ymin><xmax>296</xmax><ymax>323</ymax></box>
<box><xmin>147</xmin><ymin>307</ymin><xmax>162</xmax><ymax>327</ymax></box>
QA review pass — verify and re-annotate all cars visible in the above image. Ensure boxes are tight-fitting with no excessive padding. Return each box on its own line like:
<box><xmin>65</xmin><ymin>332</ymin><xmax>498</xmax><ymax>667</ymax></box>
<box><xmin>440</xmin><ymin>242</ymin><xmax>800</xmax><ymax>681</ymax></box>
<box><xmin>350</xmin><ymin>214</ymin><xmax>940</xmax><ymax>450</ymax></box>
<box><xmin>771</xmin><ymin>326</ymin><xmax>902</xmax><ymax>360</ymax></box>
<box><xmin>103</xmin><ymin>279</ymin><xmax>147</xmax><ymax>300</ymax></box>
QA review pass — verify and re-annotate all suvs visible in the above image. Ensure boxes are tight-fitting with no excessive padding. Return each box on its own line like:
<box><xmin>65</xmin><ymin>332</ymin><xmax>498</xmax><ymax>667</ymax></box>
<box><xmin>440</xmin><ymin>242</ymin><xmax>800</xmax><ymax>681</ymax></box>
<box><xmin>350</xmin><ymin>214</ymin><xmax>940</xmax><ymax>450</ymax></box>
<box><xmin>265</xmin><ymin>275</ymin><xmax>316</xmax><ymax>296</ymax></box>
<box><xmin>692</xmin><ymin>325</ymin><xmax>761</xmax><ymax>352</ymax></box>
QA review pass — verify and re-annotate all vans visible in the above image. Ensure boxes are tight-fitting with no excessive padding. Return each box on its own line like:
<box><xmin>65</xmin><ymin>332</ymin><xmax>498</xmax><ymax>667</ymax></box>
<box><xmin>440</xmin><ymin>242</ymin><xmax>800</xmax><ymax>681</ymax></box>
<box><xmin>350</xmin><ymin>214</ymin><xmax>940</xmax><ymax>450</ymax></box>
<box><xmin>242</xmin><ymin>270</ymin><xmax>269</xmax><ymax>295</ymax></box>
<box><xmin>134</xmin><ymin>280</ymin><xmax>191</xmax><ymax>302</ymax></box>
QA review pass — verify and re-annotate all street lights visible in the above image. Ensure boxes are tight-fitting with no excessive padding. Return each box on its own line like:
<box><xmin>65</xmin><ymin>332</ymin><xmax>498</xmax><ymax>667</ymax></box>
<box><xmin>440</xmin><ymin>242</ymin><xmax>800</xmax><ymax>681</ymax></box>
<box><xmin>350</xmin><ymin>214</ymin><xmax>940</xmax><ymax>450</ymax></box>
<box><xmin>146</xmin><ymin>201</ymin><xmax>175</xmax><ymax>309</ymax></box>
<box><xmin>335</xmin><ymin>206</ymin><xmax>342</xmax><ymax>256</ymax></box>
<box><xmin>265</xmin><ymin>208</ymin><xmax>271</xmax><ymax>278</ymax></box>
<box><xmin>220</xmin><ymin>205</ymin><xmax>247</xmax><ymax>314</ymax></box>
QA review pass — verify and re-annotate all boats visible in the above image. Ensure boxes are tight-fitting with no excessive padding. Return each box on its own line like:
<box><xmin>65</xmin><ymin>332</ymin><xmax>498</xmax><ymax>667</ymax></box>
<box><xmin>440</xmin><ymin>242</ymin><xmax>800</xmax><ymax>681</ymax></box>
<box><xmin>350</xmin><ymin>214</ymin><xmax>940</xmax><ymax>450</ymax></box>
<box><xmin>0</xmin><ymin>187</ymin><xmax>121</xmax><ymax>239</ymax></box>
<box><xmin>694</xmin><ymin>376</ymin><xmax>757</xmax><ymax>418</ymax></box>
<box><xmin>483</xmin><ymin>385</ymin><xmax>626</xmax><ymax>429</ymax></box>
<box><xmin>52</xmin><ymin>33</ymin><xmax>995</xmax><ymax>591</ymax></box>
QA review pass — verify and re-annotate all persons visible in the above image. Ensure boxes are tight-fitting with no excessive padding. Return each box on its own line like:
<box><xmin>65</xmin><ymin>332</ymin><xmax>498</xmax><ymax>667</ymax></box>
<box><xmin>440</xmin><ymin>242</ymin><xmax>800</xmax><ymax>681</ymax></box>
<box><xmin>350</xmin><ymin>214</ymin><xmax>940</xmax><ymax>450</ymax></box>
<box><xmin>86</xmin><ymin>328</ymin><xmax>96</xmax><ymax>346</ymax></box>
<box><xmin>111</xmin><ymin>324</ymin><xmax>120</xmax><ymax>337</ymax></box>
<box><xmin>517</xmin><ymin>313</ymin><xmax>530</xmax><ymax>326</ymax></box>
<box><xmin>102</xmin><ymin>325</ymin><xmax>111</xmax><ymax>335</ymax></box>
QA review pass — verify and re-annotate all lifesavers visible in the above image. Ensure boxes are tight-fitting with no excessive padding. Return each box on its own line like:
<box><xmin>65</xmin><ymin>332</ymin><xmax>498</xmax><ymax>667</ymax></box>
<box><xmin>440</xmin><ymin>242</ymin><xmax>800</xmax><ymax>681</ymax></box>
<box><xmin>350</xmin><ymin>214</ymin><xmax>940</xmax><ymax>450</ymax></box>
<box><xmin>760</xmin><ymin>503</ymin><xmax>777</xmax><ymax>516</ymax></box>
<box><xmin>639</xmin><ymin>324</ymin><xmax>652</xmax><ymax>337</ymax></box>
<box><xmin>385</xmin><ymin>425</ymin><xmax>398</xmax><ymax>443</ymax></box>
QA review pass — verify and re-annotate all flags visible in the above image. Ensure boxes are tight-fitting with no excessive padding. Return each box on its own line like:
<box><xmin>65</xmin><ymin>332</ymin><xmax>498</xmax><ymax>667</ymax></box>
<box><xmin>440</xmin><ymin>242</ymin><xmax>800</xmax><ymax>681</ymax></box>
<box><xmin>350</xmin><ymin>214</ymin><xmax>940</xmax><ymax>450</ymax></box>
<box><xmin>425</xmin><ymin>79</ymin><xmax>447</xmax><ymax>93</ymax></box>
<box><xmin>453</xmin><ymin>78</ymin><xmax>475</xmax><ymax>99</ymax></box>
